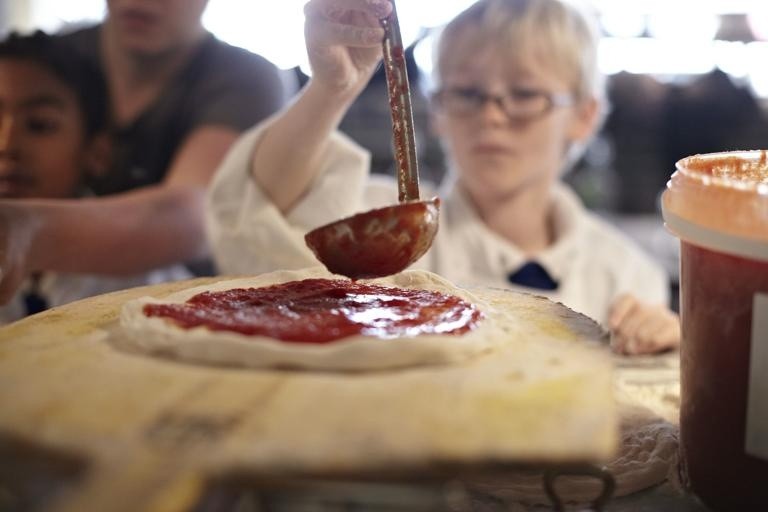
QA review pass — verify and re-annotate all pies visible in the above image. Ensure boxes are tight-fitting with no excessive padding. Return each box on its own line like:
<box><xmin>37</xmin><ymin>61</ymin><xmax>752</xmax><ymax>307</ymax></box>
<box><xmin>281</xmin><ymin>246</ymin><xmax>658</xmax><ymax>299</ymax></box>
<box><xmin>467</xmin><ymin>398</ymin><xmax>678</xmax><ymax>501</ymax></box>
<box><xmin>119</xmin><ymin>265</ymin><xmax>516</xmax><ymax>373</ymax></box>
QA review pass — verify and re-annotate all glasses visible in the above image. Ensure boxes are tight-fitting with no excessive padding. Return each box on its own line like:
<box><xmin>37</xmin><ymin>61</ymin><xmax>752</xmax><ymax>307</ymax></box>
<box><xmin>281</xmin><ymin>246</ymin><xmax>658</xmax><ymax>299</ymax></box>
<box><xmin>436</xmin><ymin>88</ymin><xmax>574</xmax><ymax>121</ymax></box>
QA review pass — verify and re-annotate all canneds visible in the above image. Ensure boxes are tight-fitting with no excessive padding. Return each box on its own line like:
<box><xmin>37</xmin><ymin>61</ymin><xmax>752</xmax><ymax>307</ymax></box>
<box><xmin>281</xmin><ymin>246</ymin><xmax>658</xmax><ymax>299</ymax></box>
<box><xmin>661</xmin><ymin>146</ymin><xmax>768</xmax><ymax>512</ymax></box>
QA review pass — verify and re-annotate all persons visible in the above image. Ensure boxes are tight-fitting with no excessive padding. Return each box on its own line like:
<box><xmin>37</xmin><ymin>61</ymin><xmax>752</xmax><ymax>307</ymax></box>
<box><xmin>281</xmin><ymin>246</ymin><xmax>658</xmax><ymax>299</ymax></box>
<box><xmin>201</xmin><ymin>1</ymin><xmax>682</xmax><ymax>360</ymax></box>
<box><xmin>0</xmin><ymin>2</ymin><xmax>286</xmax><ymax>309</ymax></box>
<box><xmin>0</xmin><ymin>31</ymin><xmax>189</xmax><ymax>323</ymax></box>
